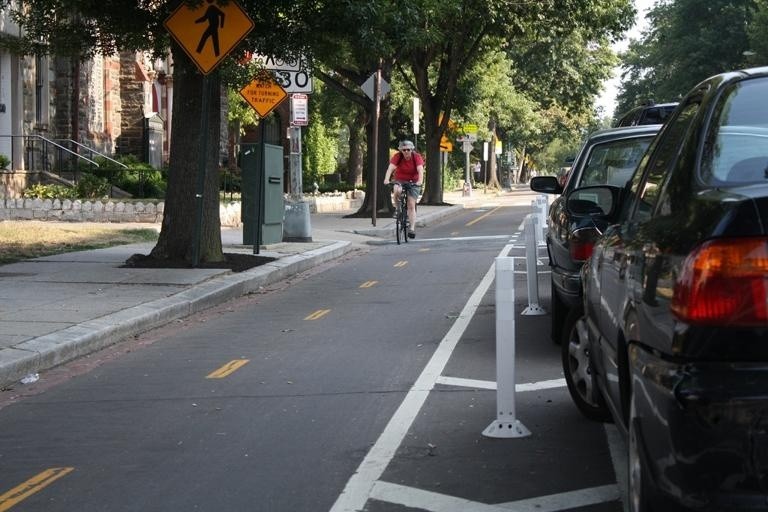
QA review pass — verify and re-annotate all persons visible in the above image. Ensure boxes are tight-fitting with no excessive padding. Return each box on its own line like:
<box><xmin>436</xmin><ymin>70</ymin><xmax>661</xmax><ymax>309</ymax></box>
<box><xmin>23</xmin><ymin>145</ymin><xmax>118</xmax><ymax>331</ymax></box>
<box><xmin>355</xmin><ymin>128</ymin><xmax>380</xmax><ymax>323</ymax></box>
<box><xmin>383</xmin><ymin>140</ymin><xmax>426</xmax><ymax>238</ymax></box>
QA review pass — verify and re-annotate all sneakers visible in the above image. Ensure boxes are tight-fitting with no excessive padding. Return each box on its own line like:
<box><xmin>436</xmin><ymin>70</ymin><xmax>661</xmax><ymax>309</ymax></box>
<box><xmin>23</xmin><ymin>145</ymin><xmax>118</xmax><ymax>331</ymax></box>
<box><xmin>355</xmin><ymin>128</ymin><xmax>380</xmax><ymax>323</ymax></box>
<box><xmin>393</xmin><ymin>209</ymin><xmax>401</xmax><ymax>218</ymax></box>
<box><xmin>407</xmin><ymin>233</ymin><xmax>416</xmax><ymax>238</ymax></box>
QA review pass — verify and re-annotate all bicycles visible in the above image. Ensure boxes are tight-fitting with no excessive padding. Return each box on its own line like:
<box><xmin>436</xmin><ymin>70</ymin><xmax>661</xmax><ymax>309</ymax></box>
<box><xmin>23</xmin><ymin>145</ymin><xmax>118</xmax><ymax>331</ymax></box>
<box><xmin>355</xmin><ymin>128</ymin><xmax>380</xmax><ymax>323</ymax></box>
<box><xmin>388</xmin><ymin>181</ymin><xmax>416</xmax><ymax>244</ymax></box>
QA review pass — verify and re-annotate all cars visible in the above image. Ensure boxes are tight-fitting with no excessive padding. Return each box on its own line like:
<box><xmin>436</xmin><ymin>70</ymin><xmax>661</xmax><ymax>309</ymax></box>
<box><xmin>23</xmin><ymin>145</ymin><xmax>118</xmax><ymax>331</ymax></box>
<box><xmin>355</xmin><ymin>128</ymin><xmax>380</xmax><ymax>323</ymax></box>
<box><xmin>614</xmin><ymin>102</ymin><xmax>680</xmax><ymax>128</ymax></box>
<box><xmin>560</xmin><ymin>65</ymin><xmax>767</xmax><ymax>510</ymax></box>
<box><xmin>530</xmin><ymin>123</ymin><xmax>768</xmax><ymax>343</ymax></box>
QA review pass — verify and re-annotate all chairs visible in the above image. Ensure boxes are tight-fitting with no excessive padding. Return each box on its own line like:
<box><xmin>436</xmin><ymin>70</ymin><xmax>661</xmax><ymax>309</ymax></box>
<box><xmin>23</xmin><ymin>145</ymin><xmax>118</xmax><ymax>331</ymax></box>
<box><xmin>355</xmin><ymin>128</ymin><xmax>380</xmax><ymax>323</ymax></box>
<box><xmin>726</xmin><ymin>156</ymin><xmax>768</xmax><ymax>184</ymax></box>
<box><xmin>601</xmin><ymin>158</ymin><xmax>639</xmax><ymax>186</ymax></box>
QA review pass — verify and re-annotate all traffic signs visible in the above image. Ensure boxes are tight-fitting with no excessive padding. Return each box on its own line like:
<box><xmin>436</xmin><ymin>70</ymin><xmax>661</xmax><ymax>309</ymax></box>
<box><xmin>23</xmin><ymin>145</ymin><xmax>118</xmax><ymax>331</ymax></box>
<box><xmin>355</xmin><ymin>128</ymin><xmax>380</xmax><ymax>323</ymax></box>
<box><xmin>277</xmin><ymin>44</ymin><xmax>313</xmax><ymax>95</ymax></box>
<box><xmin>261</xmin><ymin>42</ymin><xmax>300</xmax><ymax>72</ymax></box>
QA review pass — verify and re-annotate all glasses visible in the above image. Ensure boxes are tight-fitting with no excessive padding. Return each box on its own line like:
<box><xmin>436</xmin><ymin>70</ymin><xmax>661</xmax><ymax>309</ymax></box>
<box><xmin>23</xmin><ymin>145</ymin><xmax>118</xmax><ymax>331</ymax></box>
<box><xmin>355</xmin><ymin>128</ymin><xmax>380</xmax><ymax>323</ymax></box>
<box><xmin>402</xmin><ymin>149</ymin><xmax>410</xmax><ymax>151</ymax></box>
